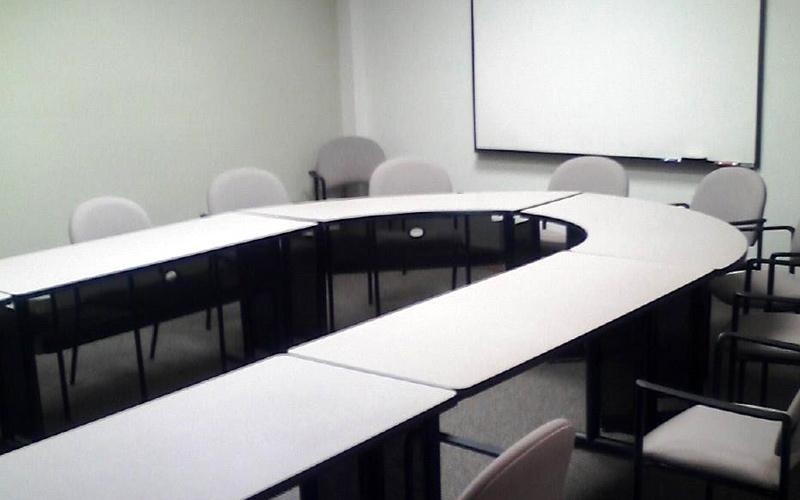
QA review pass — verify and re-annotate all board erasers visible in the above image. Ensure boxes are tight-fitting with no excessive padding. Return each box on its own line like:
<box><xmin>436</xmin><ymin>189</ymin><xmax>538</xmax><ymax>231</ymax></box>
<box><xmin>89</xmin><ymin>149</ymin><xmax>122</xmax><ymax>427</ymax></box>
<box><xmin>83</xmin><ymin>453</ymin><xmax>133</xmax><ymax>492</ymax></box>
<box><xmin>681</xmin><ymin>156</ymin><xmax>710</xmax><ymax>164</ymax></box>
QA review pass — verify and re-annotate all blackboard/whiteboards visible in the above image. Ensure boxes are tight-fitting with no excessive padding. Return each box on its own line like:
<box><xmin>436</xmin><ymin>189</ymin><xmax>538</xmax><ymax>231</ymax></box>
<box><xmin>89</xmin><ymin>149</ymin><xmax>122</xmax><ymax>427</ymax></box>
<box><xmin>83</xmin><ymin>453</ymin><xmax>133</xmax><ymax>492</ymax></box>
<box><xmin>471</xmin><ymin>0</ymin><xmax>767</xmax><ymax>170</ymax></box>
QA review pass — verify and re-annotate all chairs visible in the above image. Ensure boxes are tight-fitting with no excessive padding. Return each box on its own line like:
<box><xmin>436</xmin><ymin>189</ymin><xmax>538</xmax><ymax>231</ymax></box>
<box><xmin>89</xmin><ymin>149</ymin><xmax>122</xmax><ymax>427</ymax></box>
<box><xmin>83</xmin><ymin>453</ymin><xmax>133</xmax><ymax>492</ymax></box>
<box><xmin>71</xmin><ymin>135</ymin><xmax>799</xmax><ymax>500</ymax></box>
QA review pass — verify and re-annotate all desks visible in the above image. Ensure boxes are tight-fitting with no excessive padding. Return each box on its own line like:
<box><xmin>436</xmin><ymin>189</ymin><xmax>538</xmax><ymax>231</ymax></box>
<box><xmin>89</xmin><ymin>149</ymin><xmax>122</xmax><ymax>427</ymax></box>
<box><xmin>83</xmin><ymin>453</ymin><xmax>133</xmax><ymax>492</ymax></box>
<box><xmin>1</xmin><ymin>290</ymin><xmax>13</xmax><ymax>309</ymax></box>
<box><xmin>0</xmin><ymin>208</ymin><xmax>322</xmax><ymax>446</ymax></box>
<box><xmin>1</xmin><ymin>350</ymin><xmax>460</xmax><ymax>500</ymax></box>
